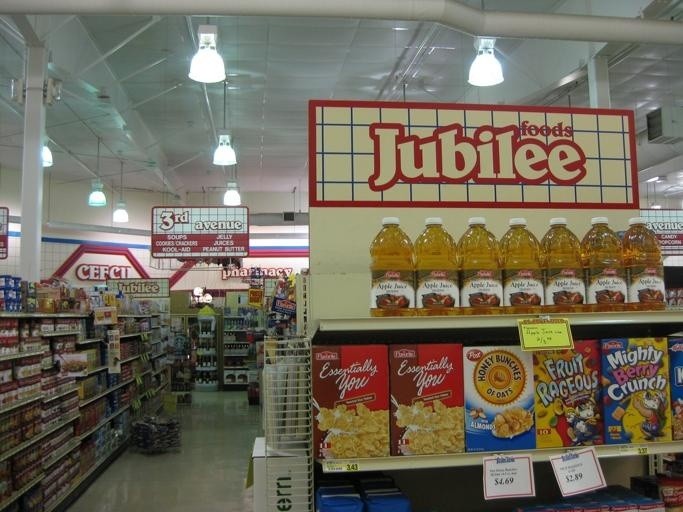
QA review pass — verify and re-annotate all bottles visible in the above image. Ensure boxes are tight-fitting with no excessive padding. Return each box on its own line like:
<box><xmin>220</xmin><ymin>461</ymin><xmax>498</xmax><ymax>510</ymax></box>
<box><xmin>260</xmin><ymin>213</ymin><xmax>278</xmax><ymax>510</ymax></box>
<box><xmin>196</xmin><ymin>338</ymin><xmax>217</xmax><ymax>352</ymax></box>
<box><xmin>414</xmin><ymin>217</ymin><xmax>459</xmax><ymax>315</ymax></box>
<box><xmin>499</xmin><ymin>217</ymin><xmax>545</xmax><ymax>313</ymax></box>
<box><xmin>580</xmin><ymin>217</ymin><xmax>628</xmax><ymax>312</ymax></box>
<box><xmin>542</xmin><ymin>217</ymin><xmax>585</xmax><ymax>312</ymax></box>
<box><xmin>194</xmin><ymin>372</ymin><xmax>218</xmax><ymax>385</ymax></box>
<box><xmin>623</xmin><ymin>217</ymin><xmax>667</xmax><ymax>311</ymax></box>
<box><xmin>458</xmin><ymin>216</ymin><xmax>502</xmax><ymax>314</ymax></box>
<box><xmin>224</xmin><ymin>318</ymin><xmax>258</xmax><ymax>330</ymax></box>
<box><xmin>195</xmin><ymin>354</ymin><xmax>218</xmax><ymax>367</ymax></box>
<box><xmin>225</xmin><ymin>343</ymin><xmax>251</xmax><ymax>351</ymax></box>
<box><xmin>369</xmin><ymin>216</ymin><xmax>417</xmax><ymax>317</ymax></box>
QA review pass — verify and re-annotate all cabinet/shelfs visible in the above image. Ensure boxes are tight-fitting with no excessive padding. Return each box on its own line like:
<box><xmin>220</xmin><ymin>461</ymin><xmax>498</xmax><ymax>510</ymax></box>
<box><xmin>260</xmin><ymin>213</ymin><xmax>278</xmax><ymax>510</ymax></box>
<box><xmin>171</xmin><ymin>299</ymin><xmax>272</xmax><ymax>403</ymax></box>
<box><xmin>272</xmin><ymin>269</ymin><xmax>683</xmax><ymax>512</ymax></box>
<box><xmin>0</xmin><ymin>302</ymin><xmax>171</xmax><ymax>512</ymax></box>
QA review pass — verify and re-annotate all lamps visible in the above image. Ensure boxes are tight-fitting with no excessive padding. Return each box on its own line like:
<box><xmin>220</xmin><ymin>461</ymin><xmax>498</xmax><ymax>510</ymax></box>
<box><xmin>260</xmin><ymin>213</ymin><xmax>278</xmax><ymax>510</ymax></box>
<box><xmin>188</xmin><ymin>24</ymin><xmax>226</xmax><ymax>82</ymax></box>
<box><xmin>223</xmin><ymin>181</ymin><xmax>242</xmax><ymax>205</ymax></box>
<box><xmin>112</xmin><ymin>202</ymin><xmax>128</xmax><ymax>221</ymax></box>
<box><xmin>465</xmin><ymin>37</ymin><xmax>505</xmax><ymax>85</ymax></box>
<box><xmin>42</xmin><ymin>147</ymin><xmax>52</xmax><ymax>167</ymax></box>
<box><xmin>89</xmin><ymin>182</ymin><xmax>104</xmax><ymax>204</ymax></box>
<box><xmin>212</xmin><ymin>133</ymin><xmax>236</xmax><ymax>167</ymax></box>
